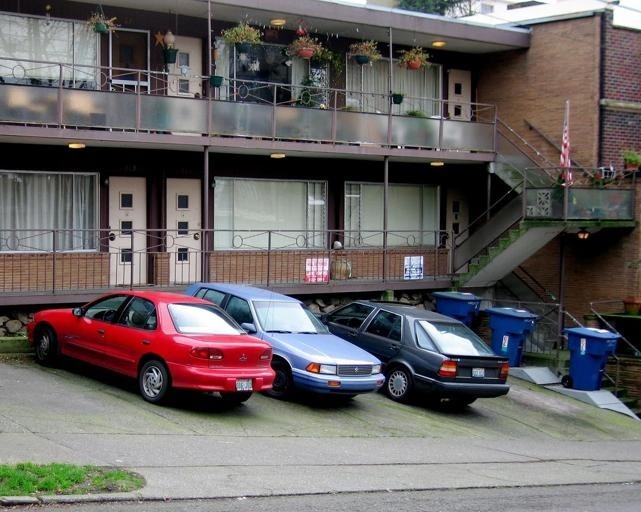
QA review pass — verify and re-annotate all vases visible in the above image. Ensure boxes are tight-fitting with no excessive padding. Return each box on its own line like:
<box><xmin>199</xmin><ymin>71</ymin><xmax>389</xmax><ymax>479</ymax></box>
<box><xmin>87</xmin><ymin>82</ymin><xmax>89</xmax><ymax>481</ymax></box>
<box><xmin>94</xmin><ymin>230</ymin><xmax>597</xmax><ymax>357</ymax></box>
<box><xmin>392</xmin><ymin>93</ymin><xmax>404</xmax><ymax>104</ymax></box>
<box><xmin>211</xmin><ymin>75</ymin><xmax>223</xmax><ymax>87</ymax></box>
<box><xmin>163</xmin><ymin>48</ymin><xmax>179</xmax><ymax>63</ymax></box>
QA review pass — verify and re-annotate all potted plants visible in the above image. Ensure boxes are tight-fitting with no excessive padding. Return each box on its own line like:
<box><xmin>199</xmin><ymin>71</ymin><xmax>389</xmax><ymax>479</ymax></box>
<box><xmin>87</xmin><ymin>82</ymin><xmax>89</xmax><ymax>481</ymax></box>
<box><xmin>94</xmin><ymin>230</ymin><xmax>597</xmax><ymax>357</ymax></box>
<box><xmin>87</xmin><ymin>11</ymin><xmax>122</xmax><ymax>35</ymax></box>
<box><xmin>347</xmin><ymin>39</ymin><xmax>382</xmax><ymax>65</ymax></box>
<box><xmin>624</xmin><ymin>150</ymin><xmax>641</xmax><ymax>171</ymax></box>
<box><xmin>223</xmin><ymin>21</ymin><xmax>264</xmax><ymax>53</ymax></box>
<box><xmin>396</xmin><ymin>46</ymin><xmax>432</xmax><ymax>71</ymax></box>
<box><xmin>284</xmin><ymin>36</ymin><xmax>322</xmax><ymax>60</ymax></box>
<box><xmin>309</xmin><ymin>47</ymin><xmax>344</xmax><ymax>73</ymax></box>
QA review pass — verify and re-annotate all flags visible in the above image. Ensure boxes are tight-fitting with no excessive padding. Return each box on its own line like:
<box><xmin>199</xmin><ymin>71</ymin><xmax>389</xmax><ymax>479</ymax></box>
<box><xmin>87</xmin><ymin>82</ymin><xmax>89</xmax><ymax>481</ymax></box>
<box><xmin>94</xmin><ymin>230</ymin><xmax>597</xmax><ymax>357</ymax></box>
<box><xmin>559</xmin><ymin>101</ymin><xmax>573</xmax><ymax>182</ymax></box>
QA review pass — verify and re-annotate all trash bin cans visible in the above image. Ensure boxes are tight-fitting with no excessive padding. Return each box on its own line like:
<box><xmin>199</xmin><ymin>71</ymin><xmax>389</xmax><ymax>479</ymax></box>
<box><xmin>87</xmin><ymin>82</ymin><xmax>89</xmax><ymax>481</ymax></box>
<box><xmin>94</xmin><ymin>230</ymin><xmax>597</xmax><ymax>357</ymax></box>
<box><xmin>561</xmin><ymin>327</ymin><xmax>622</xmax><ymax>391</ymax></box>
<box><xmin>432</xmin><ymin>291</ymin><xmax>483</xmax><ymax>331</ymax></box>
<box><xmin>485</xmin><ymin>307</ymin><xmax>539</xmax><ymax>367</ymax></box>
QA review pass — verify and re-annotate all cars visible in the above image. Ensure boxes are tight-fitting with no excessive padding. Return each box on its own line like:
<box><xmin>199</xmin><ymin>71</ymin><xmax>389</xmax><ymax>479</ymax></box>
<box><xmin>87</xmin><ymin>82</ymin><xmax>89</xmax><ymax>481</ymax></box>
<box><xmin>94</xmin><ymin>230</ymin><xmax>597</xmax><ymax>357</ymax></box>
<box><xmin>324</xmin><ymin>294</ymin><xmax>511</xmax><ymax>410</ymax></box>
<box><xmin>21</xmin><ymin>287</ymin><xmax>276</xmax><ymax>404</ymax></box>
<box><xmin>189</xmin><ymin>281</ymin><xmax>385</xmax><ymax>397</ymax></box>
